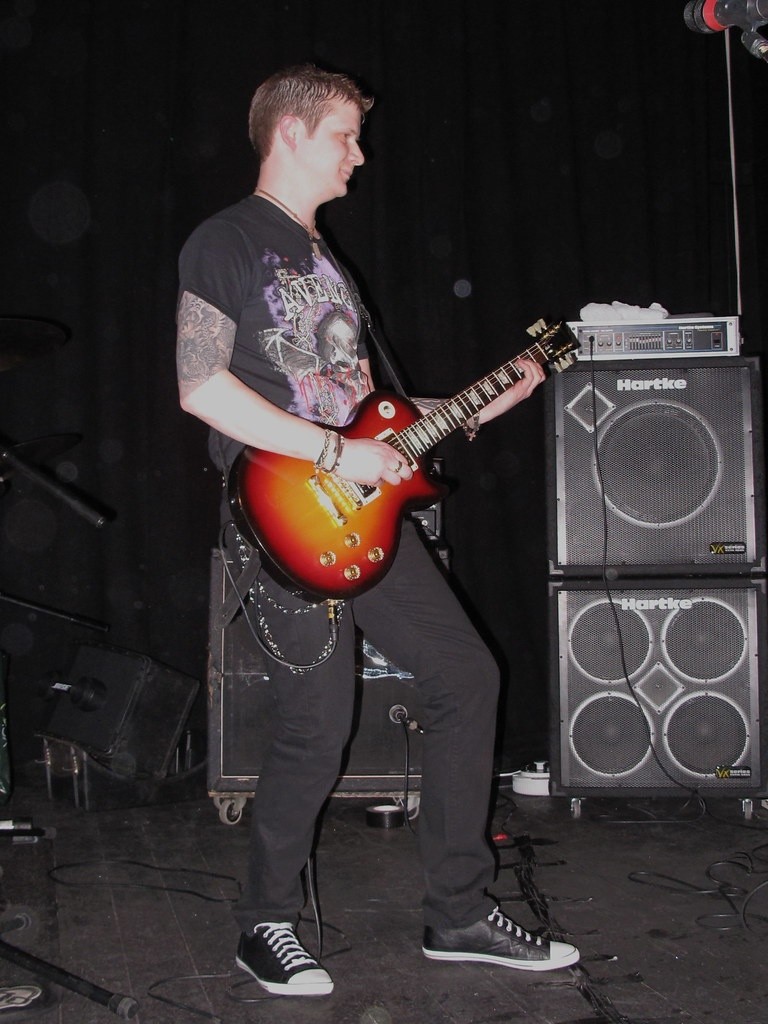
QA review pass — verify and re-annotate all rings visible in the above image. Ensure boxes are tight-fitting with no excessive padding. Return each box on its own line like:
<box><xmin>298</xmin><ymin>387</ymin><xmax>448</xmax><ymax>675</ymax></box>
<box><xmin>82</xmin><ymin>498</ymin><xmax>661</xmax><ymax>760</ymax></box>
<box><xmin>396</xmin><ymin>461</ymin><xmax>402</xmax><ymax>471</ymax></box>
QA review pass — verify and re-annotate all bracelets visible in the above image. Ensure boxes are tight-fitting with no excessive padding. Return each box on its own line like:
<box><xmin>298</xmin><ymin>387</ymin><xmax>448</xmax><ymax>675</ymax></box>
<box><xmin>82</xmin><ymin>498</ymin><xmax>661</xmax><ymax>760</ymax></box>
<box><xmin>314</xmin><ymin>429</ymin><xmax>330</xmax><ymax>468</ymax></box>
<box><xmin>463</xmin><ymin>411</ymin><xmax>479</xmax><ymax>441</ymax></box>
<box><xmin>322</xmin><ymin>433</ymin><xmax>344</xmax><ymax>474</ymax></box>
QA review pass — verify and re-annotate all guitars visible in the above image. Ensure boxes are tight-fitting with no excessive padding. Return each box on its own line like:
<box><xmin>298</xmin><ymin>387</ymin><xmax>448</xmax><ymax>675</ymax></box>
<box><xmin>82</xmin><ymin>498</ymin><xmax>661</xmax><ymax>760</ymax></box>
<box><xmin>227</xmin><ymin>315</ymin><xmax>582</xmax><ymax>607</ymax></box>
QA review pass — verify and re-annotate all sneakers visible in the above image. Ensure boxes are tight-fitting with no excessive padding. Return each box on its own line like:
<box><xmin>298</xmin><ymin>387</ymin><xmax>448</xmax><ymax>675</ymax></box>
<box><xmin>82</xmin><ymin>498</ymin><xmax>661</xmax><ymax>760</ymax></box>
<box><xmin>421</xmin><ymin>906</ymin><xmax>580</xmax><ymax>972</ymax></box>
<box><xmin>234</xmin><ymin>921</ymin><xmax>334</xmax><ymax>996</ymax></box>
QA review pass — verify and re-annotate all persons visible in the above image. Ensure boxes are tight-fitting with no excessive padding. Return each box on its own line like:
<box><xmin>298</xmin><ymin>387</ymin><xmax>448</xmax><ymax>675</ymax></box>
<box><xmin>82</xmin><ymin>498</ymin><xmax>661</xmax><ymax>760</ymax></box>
<box><xmin>175</xmin><ymin>66</ymin><xmax>581</xmax><ymax>997</ymax></box>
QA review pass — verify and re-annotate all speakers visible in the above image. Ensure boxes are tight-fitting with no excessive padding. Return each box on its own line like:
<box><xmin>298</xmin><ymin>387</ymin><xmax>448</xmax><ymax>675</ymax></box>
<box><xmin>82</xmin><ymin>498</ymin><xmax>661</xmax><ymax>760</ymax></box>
<box><xmin>210</xmin><ymin>547</ymin><xmax>449</xmax><ymax>802</ymax></box>
<box><xmin>46</xmin><ymin>637</ymin><xmax>199</xmax><ymax>780</ymax></box>
<box><xmin>547</xmin><ymin>577</ymin><xmax>768</xmax><ymax>797</ymax></box>
<box><xmin>545</xmin><ymin>356</ymin><xmax>768</xmax><ymax>579</ymax></box>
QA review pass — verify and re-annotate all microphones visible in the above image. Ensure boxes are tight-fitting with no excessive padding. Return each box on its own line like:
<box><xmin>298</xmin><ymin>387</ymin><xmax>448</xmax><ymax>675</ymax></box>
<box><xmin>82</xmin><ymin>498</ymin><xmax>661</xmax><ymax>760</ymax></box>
<box><xmin>389</xmin><ymin>704</ymin><xmax>408</xmax><ymax>724</ymax></box>
<box><xmin>70</xmin><ymin>677</ymin><xmax>106</xmax><ymax>712</ymax></box>
<box><xmin>684</xmin><ymin>0</ymin><xmax>768</xmax><ymax>34</ymax></box>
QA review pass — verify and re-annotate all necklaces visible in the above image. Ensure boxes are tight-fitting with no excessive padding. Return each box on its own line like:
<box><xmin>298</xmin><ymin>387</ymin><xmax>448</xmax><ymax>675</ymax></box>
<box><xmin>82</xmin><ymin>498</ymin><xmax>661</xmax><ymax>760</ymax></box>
<box><xmin>258</xmin><ymin>188</ymin><xmax>321</xmax><ymax>260</ymax></box>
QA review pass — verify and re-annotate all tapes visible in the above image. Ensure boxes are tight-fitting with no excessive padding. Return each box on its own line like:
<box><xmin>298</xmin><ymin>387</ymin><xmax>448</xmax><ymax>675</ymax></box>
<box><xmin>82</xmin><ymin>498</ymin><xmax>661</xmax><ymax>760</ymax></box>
<box><xmin>366</xmin><ymin>804</ymin><xmax>406</xmax><ymax>827</ymax></box>
